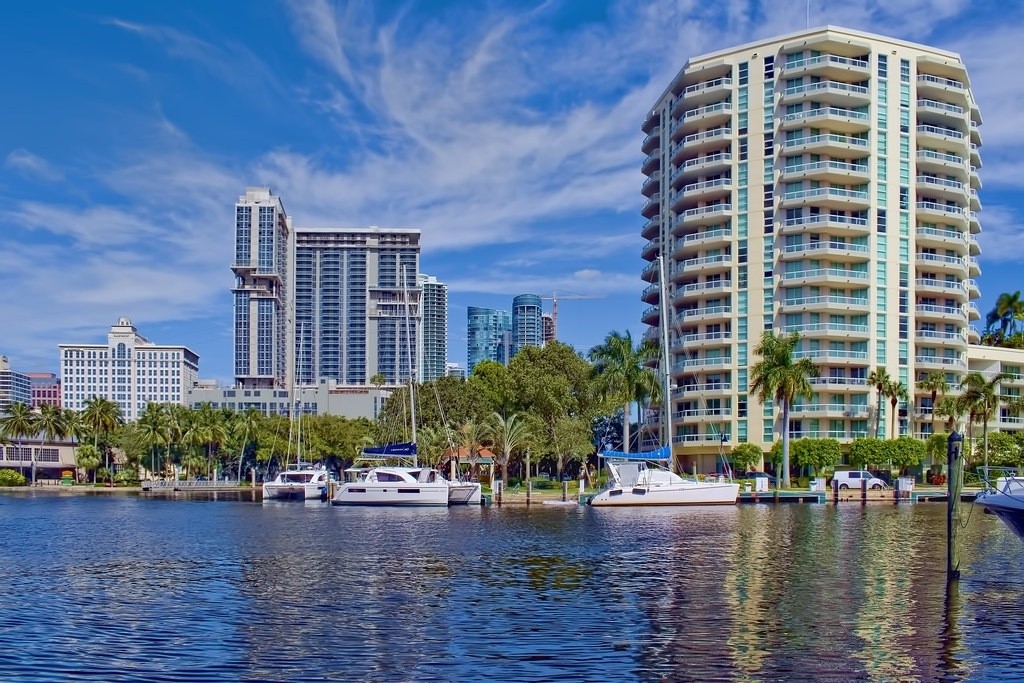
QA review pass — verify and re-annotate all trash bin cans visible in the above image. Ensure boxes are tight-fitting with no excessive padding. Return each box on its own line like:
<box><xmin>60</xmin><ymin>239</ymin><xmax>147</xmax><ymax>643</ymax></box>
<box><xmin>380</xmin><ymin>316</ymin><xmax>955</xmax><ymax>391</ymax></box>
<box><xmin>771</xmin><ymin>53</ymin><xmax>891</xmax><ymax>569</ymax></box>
<box><xmin>809</xmin><ymin>481</ymin><xmax>817</xmax><ymax>490</ymax></box>
<box><xmin>743</xmin><ymin>483</ymin><xmax>752</xmax><ymax>492</ymax></box>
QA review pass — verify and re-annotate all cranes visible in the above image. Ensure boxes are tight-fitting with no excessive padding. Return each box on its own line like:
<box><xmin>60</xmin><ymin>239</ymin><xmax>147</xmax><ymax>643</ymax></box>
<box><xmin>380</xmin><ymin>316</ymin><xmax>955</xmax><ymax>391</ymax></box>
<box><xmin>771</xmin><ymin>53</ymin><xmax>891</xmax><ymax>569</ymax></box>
<box><xmin>538</xmin><ymin>288</ymin><xmax>607</xmax><ymax>339</ymax></box>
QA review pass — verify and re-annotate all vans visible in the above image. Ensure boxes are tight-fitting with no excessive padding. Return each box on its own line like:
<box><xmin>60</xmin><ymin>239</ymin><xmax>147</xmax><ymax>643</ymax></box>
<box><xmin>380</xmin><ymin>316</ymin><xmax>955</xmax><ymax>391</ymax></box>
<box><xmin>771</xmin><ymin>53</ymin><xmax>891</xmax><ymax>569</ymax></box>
<box><xmin>831</xmin><ymin>471</ymin><xmax>884</xmax><ymax>490</ymax></box>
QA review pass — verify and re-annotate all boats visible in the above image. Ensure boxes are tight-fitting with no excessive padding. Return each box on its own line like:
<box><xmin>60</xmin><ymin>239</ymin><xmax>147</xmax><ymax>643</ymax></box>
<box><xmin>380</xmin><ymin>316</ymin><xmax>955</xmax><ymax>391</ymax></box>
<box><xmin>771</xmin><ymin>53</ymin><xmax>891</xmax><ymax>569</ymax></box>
<box><xmin>976</xmin><ymin>466</ymin><xmax>1024</xmax><ymax>547</ymax></box>
<box><xmin>331</xmin><ymin>469</ymin><xmax>449</xmax><ymax>508</ymax></box>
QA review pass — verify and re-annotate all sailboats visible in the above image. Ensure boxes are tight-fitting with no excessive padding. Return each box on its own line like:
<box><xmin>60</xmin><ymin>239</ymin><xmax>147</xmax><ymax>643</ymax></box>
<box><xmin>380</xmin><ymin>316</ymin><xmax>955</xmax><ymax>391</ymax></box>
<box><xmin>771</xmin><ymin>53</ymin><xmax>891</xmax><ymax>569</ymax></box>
<box><xmin>344</xmin><ymin>264</ymin><xmax>479</xmax><ymax>505</ymax></box>
<box><xmin>587</xmin><ymin>256</ymin><xmax>741</xmax><ymax>508</ymax></box>
<box><xmin>262</xmin><ymin>322</ymin><xmax>341</xmax><ymax>501</ymax></box>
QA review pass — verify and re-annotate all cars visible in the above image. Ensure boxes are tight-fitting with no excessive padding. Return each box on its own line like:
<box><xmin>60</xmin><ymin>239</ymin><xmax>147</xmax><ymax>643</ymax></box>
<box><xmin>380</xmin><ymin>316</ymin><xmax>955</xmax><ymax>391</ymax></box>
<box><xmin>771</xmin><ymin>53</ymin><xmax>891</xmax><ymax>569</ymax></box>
<box><xmin>745</xmin><ymin>472</ymin><xmax>782</xmax><ymax>485</ymax></box>
